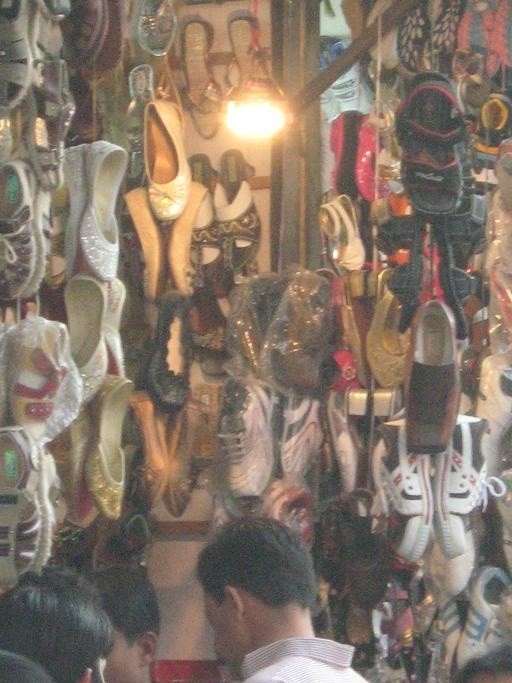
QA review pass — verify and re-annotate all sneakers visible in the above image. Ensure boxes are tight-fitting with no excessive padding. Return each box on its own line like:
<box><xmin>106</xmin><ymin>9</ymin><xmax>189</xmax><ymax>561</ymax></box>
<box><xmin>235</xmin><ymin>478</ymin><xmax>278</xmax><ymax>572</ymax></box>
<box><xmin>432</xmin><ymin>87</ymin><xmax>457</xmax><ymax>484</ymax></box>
<box><xmin>0</xmin><ymin>427</ymin><xmax>56</xmax><ymax>589</ymax></box>
<box><xmin>336</xmin><ymin>532</ymin><xmax>512</xmax><ymax>683</ymax></box>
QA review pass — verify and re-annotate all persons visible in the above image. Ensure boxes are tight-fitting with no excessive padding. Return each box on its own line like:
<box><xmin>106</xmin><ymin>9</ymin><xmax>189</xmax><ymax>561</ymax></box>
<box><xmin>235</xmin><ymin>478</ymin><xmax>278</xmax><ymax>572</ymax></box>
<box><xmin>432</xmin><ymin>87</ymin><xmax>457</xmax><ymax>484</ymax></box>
<box><xmin>451</xmin><ymin>644</ymin><xmax>511</xmax><ymax>683</ymax></box>
<box><xmin>82</xmin><ymin>565</ymin><xmax>161</xmax><ymax>681</ymax></box>
<box><xmin>1</xmin><ymin>568</ymin><xmax>115</xmax><ymax>682</ymax></box>
<box><xmin>193</xmin><ymin>515</ymin><xmax>370</xmax><ymax>683</ymax></box>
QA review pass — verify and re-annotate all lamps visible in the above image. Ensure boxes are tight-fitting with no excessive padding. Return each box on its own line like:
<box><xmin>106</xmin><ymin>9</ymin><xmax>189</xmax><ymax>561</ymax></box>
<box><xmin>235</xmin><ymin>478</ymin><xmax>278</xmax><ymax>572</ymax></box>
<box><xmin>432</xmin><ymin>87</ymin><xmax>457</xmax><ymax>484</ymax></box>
<box><xmin>222</xmin><ymin>49</ymin><xmax>289</xmax><ymax>141</ymax></box>
